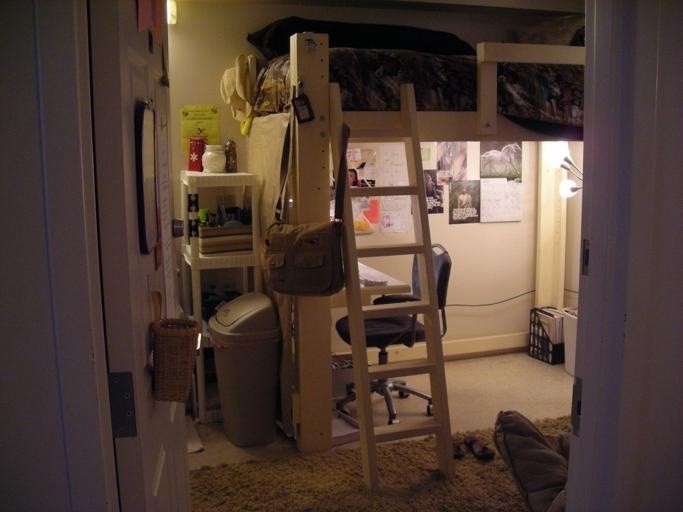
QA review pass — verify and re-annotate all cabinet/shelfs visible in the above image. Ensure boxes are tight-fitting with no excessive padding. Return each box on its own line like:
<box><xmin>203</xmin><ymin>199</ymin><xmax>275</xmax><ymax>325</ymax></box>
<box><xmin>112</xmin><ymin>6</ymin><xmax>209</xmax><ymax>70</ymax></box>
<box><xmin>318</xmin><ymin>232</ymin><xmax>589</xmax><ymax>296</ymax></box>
<box><xmin>176</xmin><ymin>170</ymin><xmax>267</xmax><ymax>428</ymax></box>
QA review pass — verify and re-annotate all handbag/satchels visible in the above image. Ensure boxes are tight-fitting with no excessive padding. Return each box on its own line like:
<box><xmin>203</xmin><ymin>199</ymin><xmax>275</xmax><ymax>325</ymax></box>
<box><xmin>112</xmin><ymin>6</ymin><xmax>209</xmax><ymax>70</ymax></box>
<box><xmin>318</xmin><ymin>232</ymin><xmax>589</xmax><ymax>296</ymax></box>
<box><xmin>265</xmin><ymin>219</ymin><xmax>343</xmax><ymax>296</ymax></box>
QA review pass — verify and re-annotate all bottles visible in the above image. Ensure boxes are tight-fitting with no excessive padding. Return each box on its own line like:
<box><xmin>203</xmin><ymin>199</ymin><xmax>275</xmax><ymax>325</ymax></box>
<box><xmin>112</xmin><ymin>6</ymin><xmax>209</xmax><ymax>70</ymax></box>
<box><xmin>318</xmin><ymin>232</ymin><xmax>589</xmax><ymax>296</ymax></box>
<box><xmin>225</xmin><ymin>138</ymin><xmax>238</xmax><ymax>173</ymax></box>
<box><xmin>225</xmin><ymin>207</ymin><xmax>241</xmax><ymax>221</ymax></box>
<box><xmin>215</xmin><ymin>203</ymin><xmax>226</xmax><ymax>226</ymax></box>
<box><xmin>200</xmin><ymin>143</ymin><xmax>226</xmax><ymax>175</ymax></box>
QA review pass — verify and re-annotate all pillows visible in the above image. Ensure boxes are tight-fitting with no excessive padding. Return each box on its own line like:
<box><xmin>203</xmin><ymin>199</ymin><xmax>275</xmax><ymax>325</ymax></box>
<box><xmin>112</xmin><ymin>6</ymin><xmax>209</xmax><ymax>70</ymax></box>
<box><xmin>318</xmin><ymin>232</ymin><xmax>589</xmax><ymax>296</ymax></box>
<box><xmin>246</xmin><ymin>16</ymin><xmax>476</xmax><ymax>63</ymax></box>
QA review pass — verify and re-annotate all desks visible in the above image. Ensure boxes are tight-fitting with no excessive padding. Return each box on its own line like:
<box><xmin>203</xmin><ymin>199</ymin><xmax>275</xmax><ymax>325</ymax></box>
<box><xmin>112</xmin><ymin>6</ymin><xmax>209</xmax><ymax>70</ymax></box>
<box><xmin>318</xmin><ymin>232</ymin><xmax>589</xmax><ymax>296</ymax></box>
<box><xmin>330</xmin><ymin>258</ymin><xmax>412</xmax><ymax>312</ymax></box>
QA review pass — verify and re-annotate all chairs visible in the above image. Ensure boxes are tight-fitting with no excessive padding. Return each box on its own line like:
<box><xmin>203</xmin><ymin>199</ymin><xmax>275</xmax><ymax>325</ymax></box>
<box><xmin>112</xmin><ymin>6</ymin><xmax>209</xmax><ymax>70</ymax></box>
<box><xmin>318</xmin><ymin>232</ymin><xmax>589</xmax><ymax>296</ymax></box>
<box><xmin>333</xmin><ymin>241</ymin><xmax>452</xmax><ymax>427</ymax></box>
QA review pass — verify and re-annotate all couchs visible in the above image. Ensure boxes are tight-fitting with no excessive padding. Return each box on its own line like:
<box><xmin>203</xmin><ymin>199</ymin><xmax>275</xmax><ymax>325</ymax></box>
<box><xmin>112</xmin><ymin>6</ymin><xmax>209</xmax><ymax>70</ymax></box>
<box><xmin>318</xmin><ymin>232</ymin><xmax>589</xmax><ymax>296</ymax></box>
<box><xmin>491</xmin><ymin>408</ymin><xmax>567</xmax><ymax>511</ymax></box>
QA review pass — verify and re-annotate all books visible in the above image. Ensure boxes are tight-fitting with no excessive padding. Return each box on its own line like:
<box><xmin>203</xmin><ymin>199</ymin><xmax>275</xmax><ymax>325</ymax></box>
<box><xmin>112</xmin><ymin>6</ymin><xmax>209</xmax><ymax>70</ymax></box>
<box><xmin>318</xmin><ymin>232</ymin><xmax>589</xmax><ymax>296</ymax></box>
<box><xmin>529</xmin><ymin>307</ymin><xmax>564</xmax><ymax>365</ymax></box>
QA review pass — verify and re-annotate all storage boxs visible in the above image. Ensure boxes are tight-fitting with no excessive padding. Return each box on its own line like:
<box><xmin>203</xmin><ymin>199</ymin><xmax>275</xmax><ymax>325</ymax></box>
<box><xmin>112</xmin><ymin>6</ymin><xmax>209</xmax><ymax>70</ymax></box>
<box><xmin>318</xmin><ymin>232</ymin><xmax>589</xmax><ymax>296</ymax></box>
<box><xmin>561</xmin><ymin>305</ymin><xmax>578</xmax><ymax>376</ymax></box>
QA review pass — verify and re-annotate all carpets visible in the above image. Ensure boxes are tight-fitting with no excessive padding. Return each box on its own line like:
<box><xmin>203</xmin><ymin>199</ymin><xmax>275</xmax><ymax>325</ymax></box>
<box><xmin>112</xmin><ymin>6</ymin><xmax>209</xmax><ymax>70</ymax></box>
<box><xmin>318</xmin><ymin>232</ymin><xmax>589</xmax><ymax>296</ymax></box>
<box><xmin>186</xmin><ymin>412</ymin><xmax>574</xmax><ymax>512</ymax></box>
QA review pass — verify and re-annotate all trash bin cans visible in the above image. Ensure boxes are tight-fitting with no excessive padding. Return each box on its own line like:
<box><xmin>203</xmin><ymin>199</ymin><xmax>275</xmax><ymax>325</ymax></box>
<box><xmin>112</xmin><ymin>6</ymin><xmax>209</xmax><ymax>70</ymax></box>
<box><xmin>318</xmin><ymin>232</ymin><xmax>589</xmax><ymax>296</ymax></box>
<box><xmin>208</xmin><ymin>292</ymin><xmax>282</xmax><ymax>447</ymax></box>
<box><xmin>563</xmin><ymin>307</ymin><xmax>579</xmax><ymax>377</ymax></box>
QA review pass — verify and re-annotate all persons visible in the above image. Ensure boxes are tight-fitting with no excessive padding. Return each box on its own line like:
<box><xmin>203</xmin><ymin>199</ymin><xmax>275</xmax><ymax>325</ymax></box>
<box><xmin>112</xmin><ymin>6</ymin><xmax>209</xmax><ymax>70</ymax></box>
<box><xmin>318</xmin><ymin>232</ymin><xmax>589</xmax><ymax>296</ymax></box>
<box><xmin>425</xmin><ymin>176</ymin><xmax>435</xmax><ymax>197</ymax></box>
<box><xmin>457</xmin><ymin>186</ymin><xmax>471</xmax><ymax>208</ymax></box>
<box><xmin>346</xmin><ymin>169</ymin><xmax>357</xmax><ymax>187</ymax></box>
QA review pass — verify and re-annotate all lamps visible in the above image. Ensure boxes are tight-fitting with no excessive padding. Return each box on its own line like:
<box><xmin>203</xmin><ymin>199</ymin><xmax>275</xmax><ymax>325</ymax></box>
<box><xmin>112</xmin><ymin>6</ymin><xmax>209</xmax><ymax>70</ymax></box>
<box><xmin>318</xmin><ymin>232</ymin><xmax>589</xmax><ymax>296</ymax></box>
<box><xmin>548</xmin><ymin>140</ymin><xmax>582</xmax><ymax>199</ymax></box>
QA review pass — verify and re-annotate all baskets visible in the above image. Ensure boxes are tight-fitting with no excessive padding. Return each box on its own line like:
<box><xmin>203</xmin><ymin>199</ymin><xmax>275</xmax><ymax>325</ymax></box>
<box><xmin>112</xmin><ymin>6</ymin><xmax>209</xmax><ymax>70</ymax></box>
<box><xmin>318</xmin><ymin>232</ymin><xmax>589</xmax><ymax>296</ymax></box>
<box><xmin>152</xmin><ymin>319</ymin><xmax>199</xmax><ymax>402</ymax></box>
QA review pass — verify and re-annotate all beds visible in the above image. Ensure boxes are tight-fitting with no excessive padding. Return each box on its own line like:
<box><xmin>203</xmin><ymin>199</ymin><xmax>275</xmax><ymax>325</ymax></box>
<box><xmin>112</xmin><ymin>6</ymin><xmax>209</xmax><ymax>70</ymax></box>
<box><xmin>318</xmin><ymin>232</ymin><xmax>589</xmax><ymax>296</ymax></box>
<box><xmin>218</xmin><ymin>31</ymin><xmax>586</xmax><ymax>457</ymax></box>
